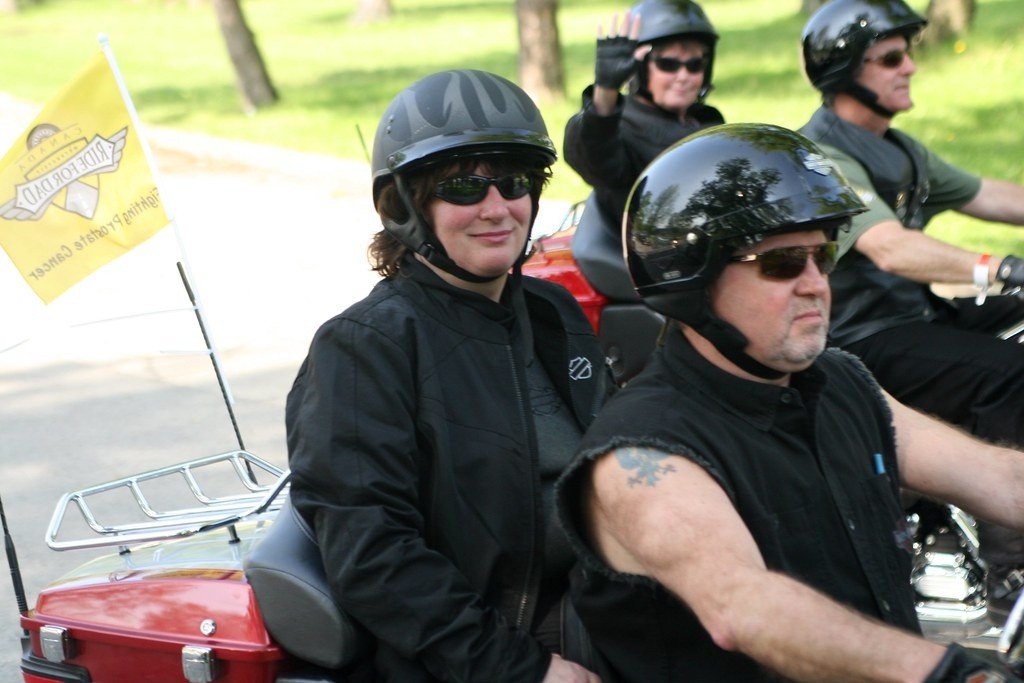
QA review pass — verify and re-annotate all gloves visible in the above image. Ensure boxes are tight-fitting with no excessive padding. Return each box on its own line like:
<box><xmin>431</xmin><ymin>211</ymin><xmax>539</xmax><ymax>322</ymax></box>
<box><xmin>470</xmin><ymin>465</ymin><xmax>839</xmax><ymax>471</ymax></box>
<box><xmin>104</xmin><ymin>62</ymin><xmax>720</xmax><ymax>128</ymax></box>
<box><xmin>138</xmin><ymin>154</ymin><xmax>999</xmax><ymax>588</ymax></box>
<box><xmin>992</xmin><ymin>255</ymin><xmax>1023</xmax><ymax>291</ymax></box>
<box><xmin>920</xmin><ymin>643</ymin><xmax>1023</xmax><ymax>683</ymax></box>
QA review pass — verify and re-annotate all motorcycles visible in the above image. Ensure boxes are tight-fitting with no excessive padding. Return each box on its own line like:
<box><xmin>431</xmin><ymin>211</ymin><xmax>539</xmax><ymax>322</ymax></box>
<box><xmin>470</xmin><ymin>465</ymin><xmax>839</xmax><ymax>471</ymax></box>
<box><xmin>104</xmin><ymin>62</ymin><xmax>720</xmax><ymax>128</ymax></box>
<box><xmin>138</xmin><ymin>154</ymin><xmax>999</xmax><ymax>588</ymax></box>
<box><xmin>501</xmin><ymin>199</ymin><xmax>1024</xmax><ymax>638</ymax></box>
<box><xmin>11</xmin><ymin>449</ymin><xmax>1024</xmax><ymax>683</ymax></box>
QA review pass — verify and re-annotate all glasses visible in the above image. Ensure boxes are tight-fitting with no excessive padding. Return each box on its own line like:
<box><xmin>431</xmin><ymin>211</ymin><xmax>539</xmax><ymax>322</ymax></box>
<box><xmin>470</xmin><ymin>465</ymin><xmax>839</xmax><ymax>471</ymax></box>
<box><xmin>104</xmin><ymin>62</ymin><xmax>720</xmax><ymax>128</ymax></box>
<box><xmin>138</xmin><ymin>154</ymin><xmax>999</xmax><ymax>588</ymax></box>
<box><xmin>861</xmin><ymin>47</ymin><xmax>910</xmax><ymax>69</ymax></box>
<box><xmin>723</xmin><ymin>240</ymin><xmax>841</xmax><ymax>281</ymax></box>
<box><xmin>648</xmin><ymin>56</ymin><xmax>709</xmax><ymax>73</ymax></box>
<box><xmin>424</xmin><ymin>173</ymin><xmax>536</xmax><ymax>206</ymax></box>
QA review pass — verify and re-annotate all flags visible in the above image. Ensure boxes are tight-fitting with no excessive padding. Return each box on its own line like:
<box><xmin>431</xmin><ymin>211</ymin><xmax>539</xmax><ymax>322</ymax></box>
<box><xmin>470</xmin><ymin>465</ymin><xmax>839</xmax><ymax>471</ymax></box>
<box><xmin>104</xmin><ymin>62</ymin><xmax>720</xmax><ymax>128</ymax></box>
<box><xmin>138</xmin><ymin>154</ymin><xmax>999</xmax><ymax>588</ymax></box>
<box><xmin>0</xmin><ymin>47</ymin><xmax>168</xmax><ymax>307</ymax></box>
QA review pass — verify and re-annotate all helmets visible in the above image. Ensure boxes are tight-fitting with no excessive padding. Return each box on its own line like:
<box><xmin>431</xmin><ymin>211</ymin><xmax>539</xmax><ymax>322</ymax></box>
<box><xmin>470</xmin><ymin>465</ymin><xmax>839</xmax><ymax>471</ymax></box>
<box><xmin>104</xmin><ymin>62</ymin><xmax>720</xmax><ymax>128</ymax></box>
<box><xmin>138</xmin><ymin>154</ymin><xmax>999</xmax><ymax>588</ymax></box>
<box><xmin>621</xmin><ymin>122</ymin><xmax>871</xmax><ymax>321</ymax></box>
<box><xmin>802</xmin><ymin>0</ymin><xmax>928</xmax><ymax>93</ymax></box>
<box><xmin>626</xmin><ymin>0</ymin><xmax>721</xmax><ymax>46</ymax></box>
<box><xmin>371</xmin><ymin>69</ymin><xmax>559</xmax><ymax>181</ymax></box>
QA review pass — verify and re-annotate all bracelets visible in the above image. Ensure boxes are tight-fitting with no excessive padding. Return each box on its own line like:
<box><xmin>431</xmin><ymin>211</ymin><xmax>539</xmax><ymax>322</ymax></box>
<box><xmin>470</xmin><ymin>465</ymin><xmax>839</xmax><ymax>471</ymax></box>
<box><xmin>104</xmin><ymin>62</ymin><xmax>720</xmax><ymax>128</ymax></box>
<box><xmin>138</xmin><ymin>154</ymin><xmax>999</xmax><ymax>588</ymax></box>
<box><xmin>973</xmin><ymin>252</ymin><xmax>993</xmax><ymax>306</ymax></box>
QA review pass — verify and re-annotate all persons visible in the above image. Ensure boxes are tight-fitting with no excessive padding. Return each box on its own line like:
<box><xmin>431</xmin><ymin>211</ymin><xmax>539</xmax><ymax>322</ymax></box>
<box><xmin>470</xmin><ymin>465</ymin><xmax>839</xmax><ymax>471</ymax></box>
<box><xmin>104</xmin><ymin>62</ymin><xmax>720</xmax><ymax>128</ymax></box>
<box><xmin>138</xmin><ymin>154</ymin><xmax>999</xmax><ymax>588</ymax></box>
<box><xmin>286</xmin><ymin>69</ymin><xmax>620</xmax><ymax>683</ymax></box>
<box><xmin>562</xmin><ymin>0</ymin><xmax>969</xmax><ymax>554</ymax></box>
<box><xmin>792</xmin><ymin>0</ymin><xmax>1024</xmax><ymax>629</ymax></box>
<box><xmin>550</xmin><ymin>123</ymin><xmax>1024</xmax><ymax>683</ymax></box>
